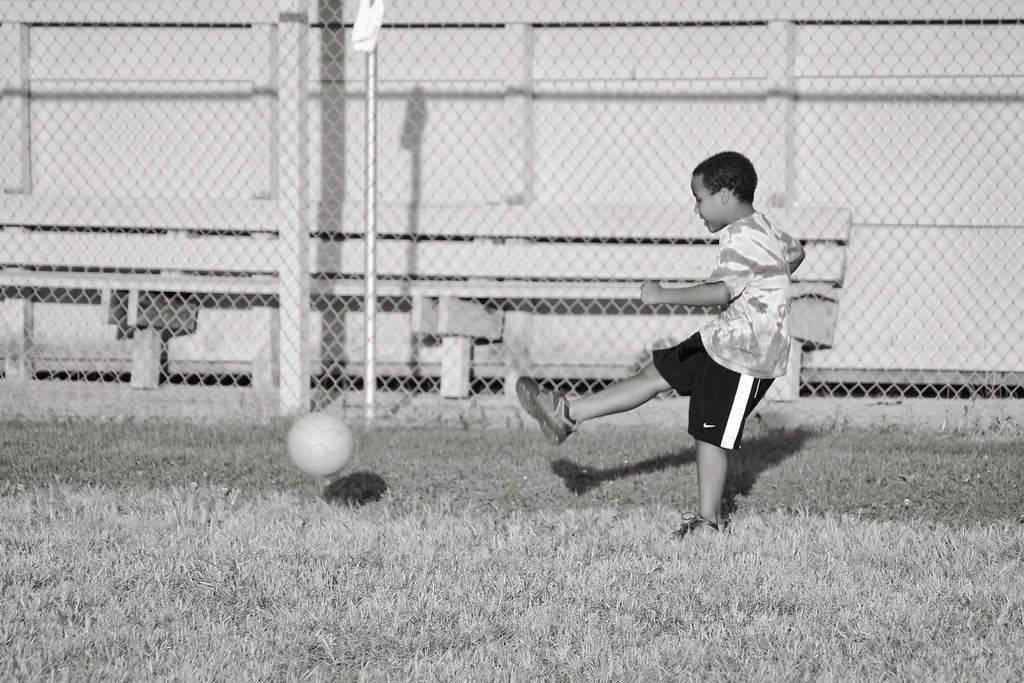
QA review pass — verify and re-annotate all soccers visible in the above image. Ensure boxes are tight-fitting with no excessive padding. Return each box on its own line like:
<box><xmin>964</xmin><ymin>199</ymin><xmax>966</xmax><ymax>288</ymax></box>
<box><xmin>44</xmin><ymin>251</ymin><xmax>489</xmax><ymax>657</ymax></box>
<box><xmin>288</xmin><ymin>413</ymin><xmax>353</xmax><ymax>477</ymax></box>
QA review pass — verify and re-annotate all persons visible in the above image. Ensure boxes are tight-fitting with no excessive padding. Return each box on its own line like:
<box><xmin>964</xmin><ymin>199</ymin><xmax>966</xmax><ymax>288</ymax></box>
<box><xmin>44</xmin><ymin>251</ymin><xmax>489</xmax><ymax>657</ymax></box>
<box><xmin>516</xmin><ymin>151</ymin><xmax>806</xmax><ymax>537</ymax></box>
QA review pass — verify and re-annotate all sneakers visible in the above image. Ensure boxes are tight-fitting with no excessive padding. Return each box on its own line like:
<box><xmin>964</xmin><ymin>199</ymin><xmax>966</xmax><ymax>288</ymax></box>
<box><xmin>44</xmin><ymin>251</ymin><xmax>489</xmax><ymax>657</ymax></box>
<box><xmin>506</xmin><ymin>373</ymin><xmax>576</xmax><ymax>446</ymax></box>
<box><xmin>671</xmin><ymin>514</ymin><xmax>733</xmax><ymax>545</ymax></box>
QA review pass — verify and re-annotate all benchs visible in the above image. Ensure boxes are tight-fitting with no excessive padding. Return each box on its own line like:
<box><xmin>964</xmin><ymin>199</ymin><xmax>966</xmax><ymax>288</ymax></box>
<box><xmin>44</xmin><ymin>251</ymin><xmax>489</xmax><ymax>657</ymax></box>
<box><xmin>0</xmin><ymin>195</ymin><xmax>853</xmax><ymax>405</ymax></box>
